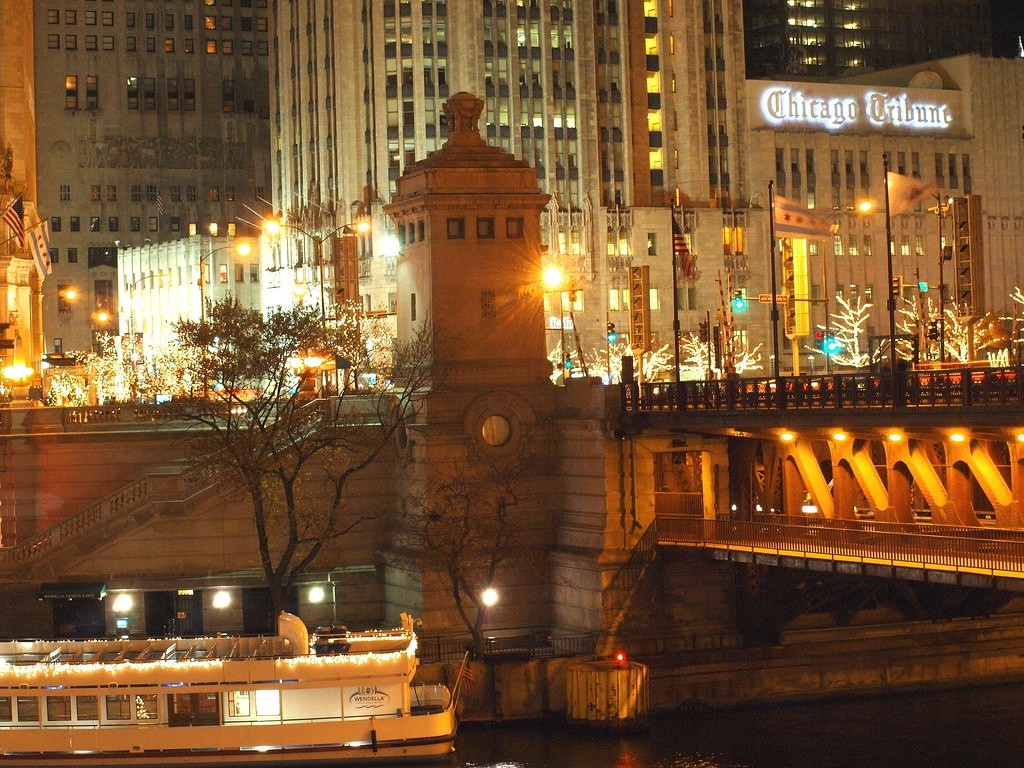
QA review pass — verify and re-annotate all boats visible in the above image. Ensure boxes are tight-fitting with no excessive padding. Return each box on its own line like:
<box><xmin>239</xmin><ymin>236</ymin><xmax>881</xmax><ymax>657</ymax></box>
<box><xmin>0</xmin><ymin>612</ymin><xmax>468</xmax><ymax>768</ymax></box>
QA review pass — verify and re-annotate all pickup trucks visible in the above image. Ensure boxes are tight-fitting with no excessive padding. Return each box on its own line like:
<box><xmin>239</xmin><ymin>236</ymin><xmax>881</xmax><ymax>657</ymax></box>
<box><xmin>45</xmin><ymin>352</ymin><xmax>90</xmax><ymax>385</ymax></box>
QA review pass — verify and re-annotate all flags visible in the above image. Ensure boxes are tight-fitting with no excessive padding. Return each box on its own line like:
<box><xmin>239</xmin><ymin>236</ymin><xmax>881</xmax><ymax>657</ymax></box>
<box><xmin>773</xmin><ymin>191</ymin><xmax>833</xmax><ymax>240</ymax></box>
<box><xmin>672</xmin><ymin>214</ymin><xmax>697</xmax><ymax>282</ymax></box>
<box><xmin>1</xmin><ymin>194</ymin><xmax>27</xmax><ymax>248</ymax></box>
<box><xmin>25</xmin><ymin>221</ymin><xmax>53</xmax><ymax>279</ymax></box>
<box><xmin>888</xmin><ymin>171</ymin><xmax>949</xmax><ymax>216</ymax></box>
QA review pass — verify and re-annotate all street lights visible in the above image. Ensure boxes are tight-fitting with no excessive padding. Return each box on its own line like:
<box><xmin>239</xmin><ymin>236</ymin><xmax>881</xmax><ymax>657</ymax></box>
<box><xmin>197</xmin><ymin>242</ymin><xmax>252</xmax><ymax>395</ymax></box>
<box><xmin>822</xmin><ymin>202</ymin><xmax>875</xmax><ymax>378</ymax></box>
<box><xmin>264</xmin><ymin>217</ymin><xmax>372</xmax><ymax>398</ymax></box>
<box><xmin>131</xmin><ymin>273</ymin><xmax>174</xmax><ymax>401</ymax></box>
<box><xmin>544</xmin><ymin>260</ymin><xmax>571</xmax><ymax>386</ymax></box>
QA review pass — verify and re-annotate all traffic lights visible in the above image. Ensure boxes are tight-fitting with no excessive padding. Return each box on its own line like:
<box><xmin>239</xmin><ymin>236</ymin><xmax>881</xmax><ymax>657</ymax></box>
<box><xmin>815</xmin><ymin>331</ymin><xmax>824</xmax><ymax>350</ymax></box>
<box><xmin>696</xmin><ymin>323</ymin><xmax>709</xmax><ymax>344</ymax></box>
<box><xmin>892</xmin><ymin>275</ymin><xmax>904</xmax><ymax>298</ymax></box>
<box><xmin>565</xmin><ymin>354</ymin><xmax>573</xmax><ymax>370</ymax></box>
<box><xmin>607</xmin><ymin>323</ymin><xmax>616</xmax><ymax>343</ymax></box>
<box><xmin>926</xmin><ymin>321</ymin><xmax>939</xmax><ymax>340</ymax></box>
<box><xmin>734</xmin><ymin>286</ymin><xmax>748</xmax><ymax>310</ymax></box>
<box><xmin>828</xmin><ymin>333</ymin><xmax>836</xmax><ymax>353</ymax></box>
